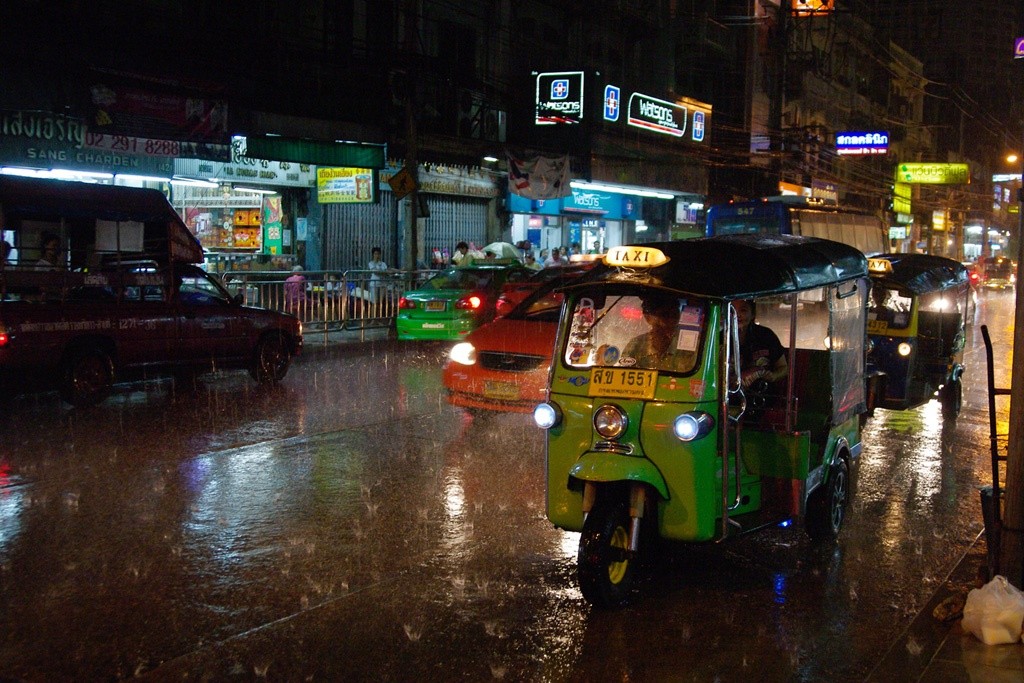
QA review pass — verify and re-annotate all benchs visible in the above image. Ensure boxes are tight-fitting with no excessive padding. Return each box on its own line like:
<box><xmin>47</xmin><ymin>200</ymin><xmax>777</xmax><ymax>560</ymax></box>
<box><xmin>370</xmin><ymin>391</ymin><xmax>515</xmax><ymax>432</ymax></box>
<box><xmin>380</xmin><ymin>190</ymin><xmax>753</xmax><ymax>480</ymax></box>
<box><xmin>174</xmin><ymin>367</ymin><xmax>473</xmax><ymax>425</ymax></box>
<box><xmin>913</xmin><ymin>313</ymin><xmax>963</xmax><ymax>368</ymax></box>
<box><xmin>782</xmin><ymin>348</ymin><xmax>833</xmax><ymax>432</ymax></box>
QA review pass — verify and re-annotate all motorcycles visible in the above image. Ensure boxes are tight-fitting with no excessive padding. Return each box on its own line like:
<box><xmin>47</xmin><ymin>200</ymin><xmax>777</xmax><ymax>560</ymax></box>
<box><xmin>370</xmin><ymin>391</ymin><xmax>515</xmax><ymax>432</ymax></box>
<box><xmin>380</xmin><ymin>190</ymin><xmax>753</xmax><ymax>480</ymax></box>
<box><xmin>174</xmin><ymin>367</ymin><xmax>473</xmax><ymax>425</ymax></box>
<box><xmin>854</xmin><ymin>251</ymin><xmax>977</xmax><ymax>418</ymax></box>
<box><xmin>535</xmin><ymin>230</ymin><xmax>874</xmax><ymax>613</ymax></box>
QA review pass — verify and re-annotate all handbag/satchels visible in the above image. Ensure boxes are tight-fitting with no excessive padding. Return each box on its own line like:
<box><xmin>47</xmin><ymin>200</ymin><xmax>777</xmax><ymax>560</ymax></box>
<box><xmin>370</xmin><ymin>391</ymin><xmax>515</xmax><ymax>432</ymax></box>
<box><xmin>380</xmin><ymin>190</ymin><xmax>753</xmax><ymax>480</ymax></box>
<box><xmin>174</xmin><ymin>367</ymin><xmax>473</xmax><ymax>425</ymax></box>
<box><xmin>747</xmin><ymin>379</ymin><xmax>786</xmax><ymax>415</ymax></box>
<box><xmin>962</xmin><ymin>575</ymin><xmax>1024</xmax><ymax>645</ymax></box>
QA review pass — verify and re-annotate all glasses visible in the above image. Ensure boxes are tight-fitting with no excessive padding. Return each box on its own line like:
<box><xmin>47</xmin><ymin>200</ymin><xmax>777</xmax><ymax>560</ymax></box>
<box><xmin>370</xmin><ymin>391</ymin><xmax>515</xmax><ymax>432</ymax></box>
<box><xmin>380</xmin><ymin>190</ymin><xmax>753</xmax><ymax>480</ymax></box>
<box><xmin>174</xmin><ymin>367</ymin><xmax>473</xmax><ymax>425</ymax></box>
<box><xmin>754</xmin><ymin>380</ymin><xmax>768</xmax><ymax>407</ymax></box>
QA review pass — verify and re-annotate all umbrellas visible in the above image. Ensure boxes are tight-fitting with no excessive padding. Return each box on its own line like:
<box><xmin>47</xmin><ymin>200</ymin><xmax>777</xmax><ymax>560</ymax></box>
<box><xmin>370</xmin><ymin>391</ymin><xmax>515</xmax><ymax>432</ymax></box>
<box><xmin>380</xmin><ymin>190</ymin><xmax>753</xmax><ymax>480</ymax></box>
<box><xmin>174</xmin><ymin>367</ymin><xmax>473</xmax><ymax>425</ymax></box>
<box><xmin>479</xmin><ymin>239</ymin><xmax>522</xmax><ymax>258</ymax></box>
<box><xmin>452</xmin><ymin>248</ymin><xmax>484</xmax><ymax>263</ymax></box>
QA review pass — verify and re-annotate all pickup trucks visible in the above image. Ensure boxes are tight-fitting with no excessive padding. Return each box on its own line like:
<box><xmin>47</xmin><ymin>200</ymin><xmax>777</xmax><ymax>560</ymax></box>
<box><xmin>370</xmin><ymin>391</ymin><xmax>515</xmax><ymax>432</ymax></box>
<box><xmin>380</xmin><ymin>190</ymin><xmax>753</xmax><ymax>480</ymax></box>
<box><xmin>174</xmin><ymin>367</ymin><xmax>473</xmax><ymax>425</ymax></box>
<box><xmin>0</xmin><ymin>251</ymin><xmax>305</xmax><ymax>408</ymax></box>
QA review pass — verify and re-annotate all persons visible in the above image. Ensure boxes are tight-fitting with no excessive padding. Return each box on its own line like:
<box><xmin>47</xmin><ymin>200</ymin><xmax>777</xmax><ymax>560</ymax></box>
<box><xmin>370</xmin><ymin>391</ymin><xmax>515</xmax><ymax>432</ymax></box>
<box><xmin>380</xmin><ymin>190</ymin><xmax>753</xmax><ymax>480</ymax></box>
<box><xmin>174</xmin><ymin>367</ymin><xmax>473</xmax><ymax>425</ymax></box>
<box><xmin>586</xmin><ymin>240</ymin><xmax>601</xmax><ymax>255</ymax></box>
<box><xmin>619</xmin><ymin>289</ymin><xmax>700</xmax><ymax>373</ymax></box>
<box><xmin>323</xmin><ymin>271</ymin><xmax>356</xmax><ymax>301</ymax></box>
<box><xmin>455</xmin><ymin>241</ymin><xmax>479</xmax><ymax>268</ymax></box>
<box><xmin>536</xmin><ymin>248</ymin><xmax>550</xmax><ymax>270</ymax></box>
<box><xmin>366</xmin><ymin>246</ymin><xmax>388</xmax><ymax>326</ymax></box>
<box><xmin>35</xmin><ymin>228</ymin><xmax>61</xmax><ymax>282</ymax></box>
<box><xmin>716</xmin><ymin>293</ymin><xmax>789</xmax><ymax>431</ymax></box>
<box><xmin>603</xmin><ymin>247</ymin><xmax>609</xmax><ymax>254</ymax></box>
<box><xmin>283</xmin><ymin>264</ymin><xmax>318</xmax><ymax>319</ymax></box>
<box><xmin>869</xmin><ymin>283</ymin><xmax>893</xmax><ymax>328</ymax></box>
<box><xmin>518</xmin><ymin>239</ymin><xmax>533</xmax><ymax>259</ymax></box>
<box><xmin>523</xmin><ymin>253</ymin><xmax>543</xmax><ymax>271</ymax></box>
<box><xmin>566</xmin><ymin>242</ymin><xmax>580</xmax><ymax>258</ymax></box>
<box><xmin>544</xmin><ymin>246</ymin><xmax>565</xmax><ymax>268</ymax></box>
<box><xmin>558</xmin><ymin>246</ymin><xmax>569</xmax><ymax>265</ymax></box>
<box><xmin>484</xmin><ymin>251</ymin><xmax>496</xmax><ymax>260</ymax></box>
<box><xmin>969</xmin><ymin>256</ymin><xmax>986</xmax><ymax>284</ymax></box>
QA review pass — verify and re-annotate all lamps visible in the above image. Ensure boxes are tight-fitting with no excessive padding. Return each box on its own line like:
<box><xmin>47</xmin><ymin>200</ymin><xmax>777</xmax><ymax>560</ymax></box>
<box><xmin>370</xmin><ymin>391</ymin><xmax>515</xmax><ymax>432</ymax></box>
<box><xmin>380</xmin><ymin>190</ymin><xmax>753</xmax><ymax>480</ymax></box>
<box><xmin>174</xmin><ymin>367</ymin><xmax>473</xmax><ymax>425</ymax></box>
<box><xmin>483</xmin><ymin>151</ymin><xmax>499</xmax><ymax>163</ymax></box>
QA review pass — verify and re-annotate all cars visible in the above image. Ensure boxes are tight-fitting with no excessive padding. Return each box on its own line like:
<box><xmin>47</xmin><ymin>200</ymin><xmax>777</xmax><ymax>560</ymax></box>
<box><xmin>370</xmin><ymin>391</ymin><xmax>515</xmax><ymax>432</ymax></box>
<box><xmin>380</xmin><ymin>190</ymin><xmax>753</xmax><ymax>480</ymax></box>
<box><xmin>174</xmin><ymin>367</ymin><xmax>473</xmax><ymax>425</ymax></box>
<box><xmin>442</xmin><ymin>270</ymin><xmax>588</xmax><ymax>416</ymax></box>
<box><xmin>495</xmin><ymin>263</ymin><xmax>593</xmax><ymax>324</ymax></box>
<box><xmin>959</xmin><ymin>255</ymin><xmax>1016</xmax><ymax>295</ymax></box>
<box><xmin>393</xmin><ymin>257</ymin><xmax>538</xmax><ymax>347</ymax></box>
